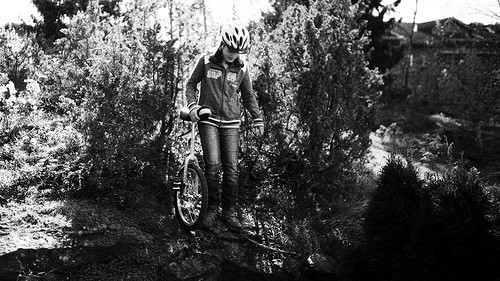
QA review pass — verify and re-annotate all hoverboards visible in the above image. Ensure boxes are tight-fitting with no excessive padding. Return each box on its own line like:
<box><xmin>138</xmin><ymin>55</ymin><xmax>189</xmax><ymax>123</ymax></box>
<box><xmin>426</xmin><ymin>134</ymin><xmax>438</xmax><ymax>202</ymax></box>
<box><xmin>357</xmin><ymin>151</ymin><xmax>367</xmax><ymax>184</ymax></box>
<box><xmin>170</xmin><ymin>108</ymin><xmax>212</xmax><ymax>231</ymax></box>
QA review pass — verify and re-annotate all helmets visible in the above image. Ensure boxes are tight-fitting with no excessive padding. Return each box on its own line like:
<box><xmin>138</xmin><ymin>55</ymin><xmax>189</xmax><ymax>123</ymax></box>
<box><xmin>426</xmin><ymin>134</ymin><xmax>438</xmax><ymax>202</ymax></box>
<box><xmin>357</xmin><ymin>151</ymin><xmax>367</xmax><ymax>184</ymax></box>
<box><xmin>221</xmin><ymin>25</ymin><xmax>250</xmax><ymax>54</ymax></box>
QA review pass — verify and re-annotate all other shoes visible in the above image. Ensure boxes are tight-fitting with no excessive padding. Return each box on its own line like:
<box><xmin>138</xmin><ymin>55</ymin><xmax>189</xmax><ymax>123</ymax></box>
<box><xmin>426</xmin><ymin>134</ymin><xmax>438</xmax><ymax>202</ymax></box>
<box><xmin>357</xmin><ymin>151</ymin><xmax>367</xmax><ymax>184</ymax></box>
<box><xmin>203</xmin><ymin>205</ymin><xmax>218</xmax><ymax>229</ymax></box>
<box><xmin>221</xmin><ymin>207</ymin><xmax>242</xmax><ymax>231</ymax></box>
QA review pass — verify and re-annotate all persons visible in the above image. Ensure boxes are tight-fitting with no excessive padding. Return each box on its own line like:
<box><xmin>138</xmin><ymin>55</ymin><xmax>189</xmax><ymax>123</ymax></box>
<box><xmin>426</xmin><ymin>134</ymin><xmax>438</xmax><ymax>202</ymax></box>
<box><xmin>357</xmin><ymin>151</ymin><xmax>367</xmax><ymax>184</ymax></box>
<box><xmin>185</xmin><ymin>24</ymin><xmax>266</xmax><ymax>234</ymax></box>
